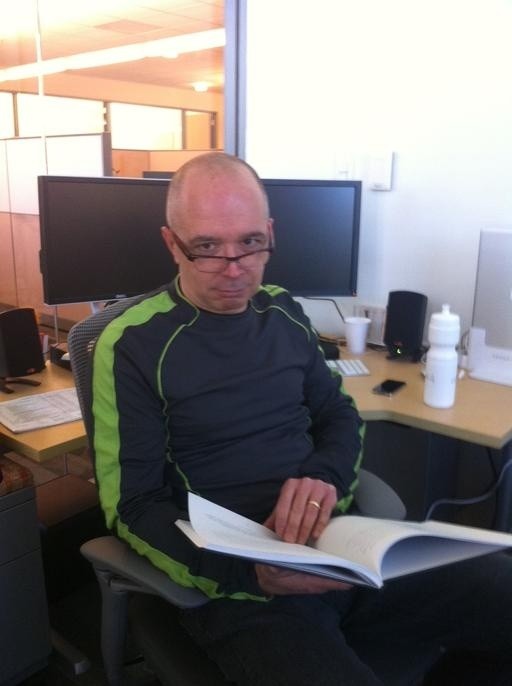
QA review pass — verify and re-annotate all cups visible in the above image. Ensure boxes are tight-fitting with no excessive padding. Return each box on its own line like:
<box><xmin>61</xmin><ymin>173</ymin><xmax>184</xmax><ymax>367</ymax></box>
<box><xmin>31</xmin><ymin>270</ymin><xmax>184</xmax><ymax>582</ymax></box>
<box><xmin>345</xmin><ymin>317</ymin><xmax>371</xmax><ymax>354</ymax></box>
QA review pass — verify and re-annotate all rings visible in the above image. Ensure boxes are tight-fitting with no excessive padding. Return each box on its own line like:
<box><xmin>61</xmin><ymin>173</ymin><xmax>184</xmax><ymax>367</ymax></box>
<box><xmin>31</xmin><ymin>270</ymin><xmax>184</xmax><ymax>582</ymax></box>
<box><xmin>308</xmin><ymin>501</ymin><xmax>321</xmax><ymax>509</ymax></box>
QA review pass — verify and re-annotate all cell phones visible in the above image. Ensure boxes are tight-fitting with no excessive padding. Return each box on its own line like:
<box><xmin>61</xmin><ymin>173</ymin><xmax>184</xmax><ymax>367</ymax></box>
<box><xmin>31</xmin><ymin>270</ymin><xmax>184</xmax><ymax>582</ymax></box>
<box><xmin>373</xmin><ymin>380</ymin><xmax>406</xmax><ymax>396</ymax></box>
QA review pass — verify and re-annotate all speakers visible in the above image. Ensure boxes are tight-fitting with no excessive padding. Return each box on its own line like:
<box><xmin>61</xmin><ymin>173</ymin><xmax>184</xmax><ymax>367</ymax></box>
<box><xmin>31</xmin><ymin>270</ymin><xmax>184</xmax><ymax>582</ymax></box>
<box><xmin>383</xmin><ymin>290</ymin><xmax>427</xmax><ymax>362</ymax></box>
<box><xmin>0</xmin><ymin>307</ymin><xmax>45</xmax><ymax>394</ymax></box>
<box><xmin>466</xmin><ymin>229</ymin><xmax>512</xmax><ymax>386</ymax></box>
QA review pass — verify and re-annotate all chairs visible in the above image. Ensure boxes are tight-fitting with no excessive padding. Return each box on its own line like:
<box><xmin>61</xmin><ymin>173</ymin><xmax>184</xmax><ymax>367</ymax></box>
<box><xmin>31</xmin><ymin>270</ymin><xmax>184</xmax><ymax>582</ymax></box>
<box><xmin>67</xmin><ymin>286</ymin><xmax>408</xmax><ymax>684</ymax></box>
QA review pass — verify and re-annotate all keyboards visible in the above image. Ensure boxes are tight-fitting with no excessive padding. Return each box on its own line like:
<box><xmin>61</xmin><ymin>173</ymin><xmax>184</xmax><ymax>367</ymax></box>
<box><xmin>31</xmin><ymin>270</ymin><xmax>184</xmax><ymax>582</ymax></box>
<box><xmin>324</xmin><ymin>359</ymin><xmax>371</xmax><ymax>377</ymax></box>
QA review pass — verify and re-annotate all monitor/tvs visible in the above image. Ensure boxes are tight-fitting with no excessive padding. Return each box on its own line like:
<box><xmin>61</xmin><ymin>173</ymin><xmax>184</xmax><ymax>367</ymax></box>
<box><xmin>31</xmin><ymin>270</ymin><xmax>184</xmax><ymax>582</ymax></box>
<box><xmin>258</xmin><ymin>178</ymin><xmax>362</xmax><ymax>297</ymax></box>
<box><xmin>37</xmin><ymin>175</ymin><xmax>179</xmax><ymax>305</ymax></box>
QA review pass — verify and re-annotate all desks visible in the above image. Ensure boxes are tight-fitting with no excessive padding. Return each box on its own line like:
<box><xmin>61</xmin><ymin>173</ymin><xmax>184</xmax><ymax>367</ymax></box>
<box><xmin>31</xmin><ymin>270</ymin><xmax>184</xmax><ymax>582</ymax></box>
<box><xmin>0</xmin><ymin>346</ymin><xmax>510</xmax><ymax>674</ymax></box>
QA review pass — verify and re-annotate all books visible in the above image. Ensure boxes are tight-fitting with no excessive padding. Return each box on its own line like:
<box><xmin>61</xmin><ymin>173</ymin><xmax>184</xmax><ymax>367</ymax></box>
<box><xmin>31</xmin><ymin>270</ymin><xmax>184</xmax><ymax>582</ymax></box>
<box><xmin>173</xmin><ymin>492</ymin><xmax>512</xmax><ymax>591</ymax></box>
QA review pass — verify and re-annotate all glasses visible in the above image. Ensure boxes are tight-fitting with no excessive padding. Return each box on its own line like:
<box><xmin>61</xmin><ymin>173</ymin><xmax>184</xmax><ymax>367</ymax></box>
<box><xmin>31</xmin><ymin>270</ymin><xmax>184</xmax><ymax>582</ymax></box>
<box><xmin>165</xmin><ymin>225</ymin><xmax>273</xmax><ymax>273</ymax></box>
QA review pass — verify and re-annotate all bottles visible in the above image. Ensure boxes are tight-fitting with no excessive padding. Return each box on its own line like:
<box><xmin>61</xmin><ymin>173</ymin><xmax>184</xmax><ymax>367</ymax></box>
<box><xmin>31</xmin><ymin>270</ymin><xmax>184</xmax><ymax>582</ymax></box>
<box><xmin>424</xmin><ymin>304</ymin><xmax>461</xmax><ymax>407</ymax></box>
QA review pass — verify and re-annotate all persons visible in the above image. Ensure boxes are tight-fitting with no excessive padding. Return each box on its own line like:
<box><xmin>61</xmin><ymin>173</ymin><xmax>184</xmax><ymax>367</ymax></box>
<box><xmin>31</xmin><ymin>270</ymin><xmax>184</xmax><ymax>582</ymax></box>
<box><xmin>88</xmin><ymin>153</ymin><xmax>512</xmax><ymax>686</ymax></box>
